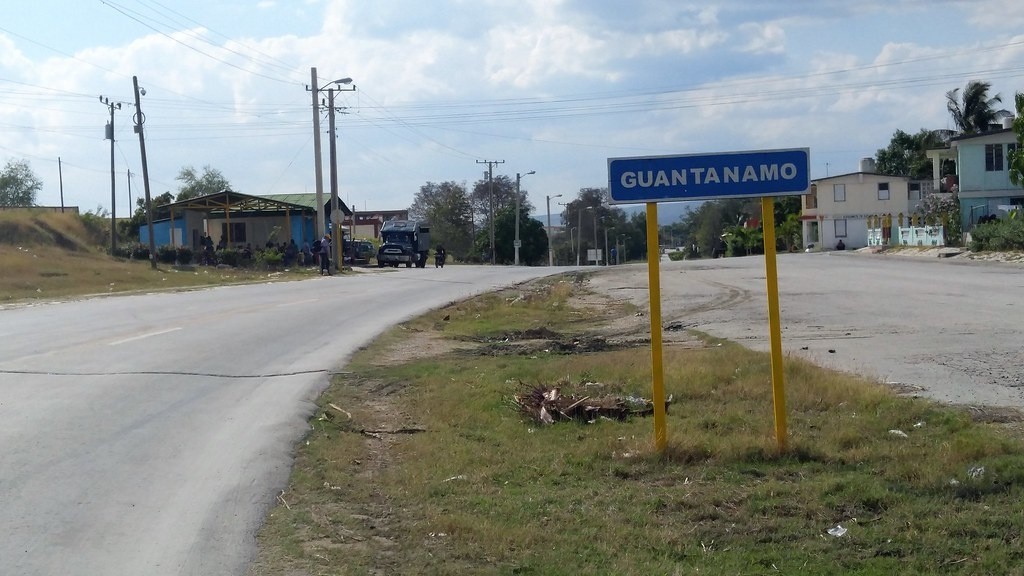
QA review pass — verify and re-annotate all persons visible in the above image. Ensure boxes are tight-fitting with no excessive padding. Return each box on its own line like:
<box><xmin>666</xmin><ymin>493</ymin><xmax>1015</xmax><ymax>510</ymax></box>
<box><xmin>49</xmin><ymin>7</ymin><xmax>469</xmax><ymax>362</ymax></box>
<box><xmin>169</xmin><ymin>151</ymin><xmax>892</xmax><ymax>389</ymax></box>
<box><xmin>219</xmin><ymin>235</ymin><xmax>226</xmax><ymax>250</ymax></box>
<box><xmin>320</xmin><ymin>235</ymin><xmax>332</xmax><ymax>275</ymax></box>
<box><xmin>205</xmin><ymin>236</ymin><xmax>213</xmax><ymax>253</ymax></box>
<box><xmin>266</xmin><ymin>237</ymin><xmax>311</xmax><ymax>267</ymax></box>
<box><xmin>342</xmin><ymin>231</ymin><xmax>346</xmax><ymax>265</ymax></box>
<box><xmin>611</xmin><ymin>247</ymin><xmax>617</xmax><ymax>264</ymax></box>
<box><xmin>837</xmin><ymin>240</ymin><xmax>845</xmax><ymax>250</ymax></box>
<box><xmin>435</xmin><ymin>243</ymin><xmax>446</xmax><ymax>265</ymax></box>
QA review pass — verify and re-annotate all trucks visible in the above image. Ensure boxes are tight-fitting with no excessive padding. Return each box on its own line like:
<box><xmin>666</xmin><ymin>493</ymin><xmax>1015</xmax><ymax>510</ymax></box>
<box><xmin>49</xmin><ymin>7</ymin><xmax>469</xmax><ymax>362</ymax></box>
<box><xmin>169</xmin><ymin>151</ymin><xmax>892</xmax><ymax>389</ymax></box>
<box><xmin>377</xmin><ymin>219</ymin><xmax>431</xmax><ymax>269</ymax></box>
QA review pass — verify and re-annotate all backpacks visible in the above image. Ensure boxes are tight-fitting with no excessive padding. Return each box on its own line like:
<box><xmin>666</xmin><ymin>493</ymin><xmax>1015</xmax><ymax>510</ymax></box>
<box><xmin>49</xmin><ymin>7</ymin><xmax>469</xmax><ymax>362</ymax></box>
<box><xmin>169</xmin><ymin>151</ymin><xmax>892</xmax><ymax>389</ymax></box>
<box><xmin>311</xmin><ymin>240</ymin><xmax>323</xmax><ymax>253</ymax></box>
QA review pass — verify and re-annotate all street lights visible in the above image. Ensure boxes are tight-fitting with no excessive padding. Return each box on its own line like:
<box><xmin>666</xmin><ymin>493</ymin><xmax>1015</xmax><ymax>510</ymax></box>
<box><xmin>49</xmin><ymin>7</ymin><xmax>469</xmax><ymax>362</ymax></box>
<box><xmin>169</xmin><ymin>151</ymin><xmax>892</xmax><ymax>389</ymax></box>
<box><xmin>515</xmin><ymin>170</ymin><xmax>536</xmax><ymax>265</ymax></box>
<box><xmin>576</xmin><ymin>205</ymin><xmax>592</xmax><ymax>266</ymax></box>
<box><xmin>593</xmin><ymin>215</ymin><xmax>632</xmax><ymax>266</ymax></box>
<box><xmin>546</xmin><ymin>194</ymin><xmax>563</xmax><ymax>266</ymax></box>
<box><xmin>311</xmin><ymin>66</ymin><xmax>353</xmax><ymax>275</ymax></box>
<box><xmin>131</xmin><ymin>76</ymin><xmax>157</xmax><ymax>268</ymax></box>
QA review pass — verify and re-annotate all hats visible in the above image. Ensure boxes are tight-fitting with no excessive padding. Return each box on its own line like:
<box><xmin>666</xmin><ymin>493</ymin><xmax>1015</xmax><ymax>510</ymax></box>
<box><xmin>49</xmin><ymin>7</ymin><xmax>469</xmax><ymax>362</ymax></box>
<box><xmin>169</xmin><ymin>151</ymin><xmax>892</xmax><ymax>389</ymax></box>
<box><xmin>324</xmin><ymin>234</ymin><xmax>332</xmax><ymax>240</ymax></box>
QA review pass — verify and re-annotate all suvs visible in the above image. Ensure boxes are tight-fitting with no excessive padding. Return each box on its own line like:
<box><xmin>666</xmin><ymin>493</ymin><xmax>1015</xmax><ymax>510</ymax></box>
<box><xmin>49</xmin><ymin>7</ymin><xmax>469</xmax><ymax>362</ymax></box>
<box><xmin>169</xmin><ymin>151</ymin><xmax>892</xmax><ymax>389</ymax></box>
<box><xmin>345</xmin><ymin>239</ymin><xmax>376</xmax><ymax>263</ymax></box>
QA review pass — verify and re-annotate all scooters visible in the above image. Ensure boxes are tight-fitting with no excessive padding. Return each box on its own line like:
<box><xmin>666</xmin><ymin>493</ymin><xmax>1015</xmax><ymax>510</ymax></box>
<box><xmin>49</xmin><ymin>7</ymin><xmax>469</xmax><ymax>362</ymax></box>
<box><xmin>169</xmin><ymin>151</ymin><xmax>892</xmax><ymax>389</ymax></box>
<box><xmin>434</xmin><ymin>253</ymin><xmax>446</xmax><ymax>268</ymax></box>
